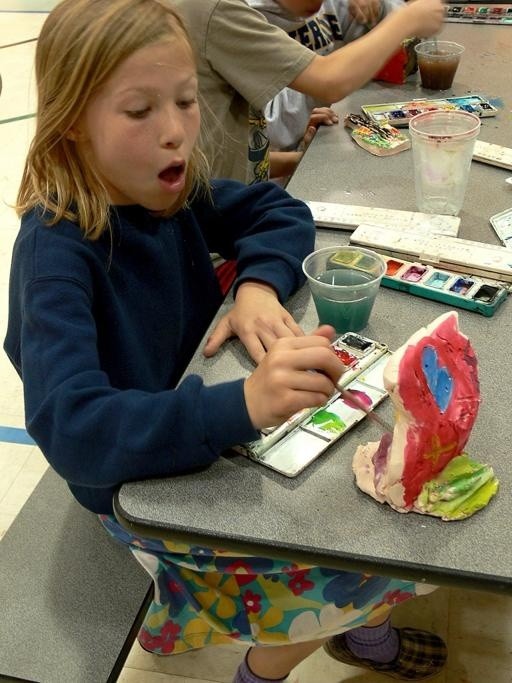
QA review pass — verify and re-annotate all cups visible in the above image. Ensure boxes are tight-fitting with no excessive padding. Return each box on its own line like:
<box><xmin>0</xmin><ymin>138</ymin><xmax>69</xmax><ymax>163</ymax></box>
<box><xmin>408</xmin><ymin>109</ymin><xmax>481</xmax><ymax>216</ymax></box>
<box><xmin>301</xmin><ymin>245</ymin><xmax>387</xmax><ymax>334</ymax></box>
<box><xmin>414</xmin><ymin>40</ymin><xmax>465</xmax><ymax>90</ymax></box>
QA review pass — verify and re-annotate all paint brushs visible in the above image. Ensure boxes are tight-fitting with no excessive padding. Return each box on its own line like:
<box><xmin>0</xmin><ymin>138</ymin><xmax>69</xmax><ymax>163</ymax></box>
<box><xmin>433</xmin><ymin>34</ymin><xmax>441</xmax><ymax>56</ymax></box>
<box><xmin>326</xmin><ymin>372</ymin><xmax>394</xmax><ymax>434</ymax></box>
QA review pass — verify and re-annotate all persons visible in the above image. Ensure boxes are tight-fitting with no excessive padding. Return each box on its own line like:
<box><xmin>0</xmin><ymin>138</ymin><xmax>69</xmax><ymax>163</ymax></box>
<box><xmin>242</xmin><ymin>2</ymin><xmax>419</xmax><ymax>190</ymax></box>
<box><xmin>155</xmin><ymin>2</ymin><xmax>449</xmax><ymax>296</ymax></box>
<box><xmin>1</xmin><ymin>1</ymin><xmax>448</xmax><ymax>683</ymax></box>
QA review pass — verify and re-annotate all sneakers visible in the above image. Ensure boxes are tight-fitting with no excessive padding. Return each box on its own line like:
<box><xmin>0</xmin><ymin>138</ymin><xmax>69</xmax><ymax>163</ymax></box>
<box><xmin>323</xmin><ymin>627</ymin><xmax>447</xmax><ymax>681</ymax></box>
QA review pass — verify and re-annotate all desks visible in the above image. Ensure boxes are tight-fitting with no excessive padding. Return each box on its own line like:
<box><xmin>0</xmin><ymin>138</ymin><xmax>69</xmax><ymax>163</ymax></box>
<box><xmin>114</xmin><ymin>23</ymin><xmax>511</xmax><ymax>598</ymax></box>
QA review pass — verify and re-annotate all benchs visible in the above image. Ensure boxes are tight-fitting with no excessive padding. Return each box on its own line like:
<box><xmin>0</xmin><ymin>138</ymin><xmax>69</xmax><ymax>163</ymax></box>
<box><xmin>0</xmin><ymin>466</ymin><xmax>154</xmax><ymax>683</ymax></box>
